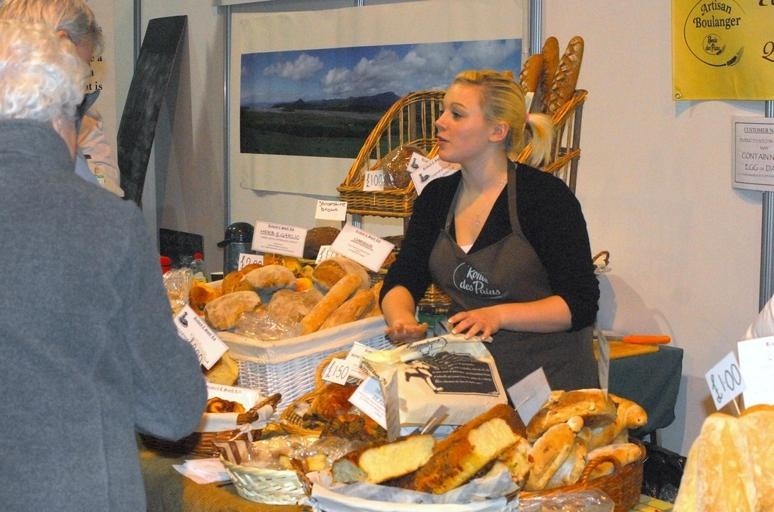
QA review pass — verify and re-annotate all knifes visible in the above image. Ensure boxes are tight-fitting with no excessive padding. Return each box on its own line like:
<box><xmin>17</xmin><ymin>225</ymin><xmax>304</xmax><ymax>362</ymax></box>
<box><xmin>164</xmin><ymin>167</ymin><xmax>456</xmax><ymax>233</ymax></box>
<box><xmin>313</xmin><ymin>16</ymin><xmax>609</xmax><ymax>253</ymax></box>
<box><xmin>590</xmin><ymin>334</ymin><xmax>672</xmax><ymax>344</ymax></box>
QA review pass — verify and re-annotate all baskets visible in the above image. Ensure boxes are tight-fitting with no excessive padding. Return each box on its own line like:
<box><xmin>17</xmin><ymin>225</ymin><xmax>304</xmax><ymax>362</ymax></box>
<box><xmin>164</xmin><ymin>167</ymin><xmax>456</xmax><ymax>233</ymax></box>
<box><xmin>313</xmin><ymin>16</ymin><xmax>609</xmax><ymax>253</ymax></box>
<box><xmin>218</xmin><ymin>306</ymin><xmax>434</xmax><ymax>435</ymax></box>
<box><xmin>337</xmin><ymin>90</ymin><xmax>588</xmax><ymax>217</ymax></box>
<box><xmin>221</xmin><ymin>382</ymin><xmax>647</xmax><ymax>511</ymax></box>
<box><xmin>139</xmin><ymin>382</ymin><xmax>647</xmax><ymax>511</ymax></box>
<box><xmin>297</xmin><ymin>227</ymin><xmax>454</xmax><ymax>312</ymax></box>
<box><xmin>140</xmin><ymin>431</ymin><xmax>241</xmax><ymax>459</ymax></box>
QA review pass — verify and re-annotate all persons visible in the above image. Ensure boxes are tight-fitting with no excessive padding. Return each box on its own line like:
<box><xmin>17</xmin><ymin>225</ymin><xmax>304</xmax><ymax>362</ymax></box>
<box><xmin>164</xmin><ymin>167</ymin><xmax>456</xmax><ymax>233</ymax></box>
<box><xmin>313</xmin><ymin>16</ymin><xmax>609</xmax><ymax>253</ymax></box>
<box><xmin>379</xmin><ymin>69</ymin><xmax>601</xmax><ymax>396</ymax></box>
<box><xmin>0</xmin><ymin>1</ymin><xmax>121</xmax><ymax>193</ymax></box>
<box><xmin>0</xmin><ymin>18</ymin><xmax>209</xmax><ymax>512</ymax></box>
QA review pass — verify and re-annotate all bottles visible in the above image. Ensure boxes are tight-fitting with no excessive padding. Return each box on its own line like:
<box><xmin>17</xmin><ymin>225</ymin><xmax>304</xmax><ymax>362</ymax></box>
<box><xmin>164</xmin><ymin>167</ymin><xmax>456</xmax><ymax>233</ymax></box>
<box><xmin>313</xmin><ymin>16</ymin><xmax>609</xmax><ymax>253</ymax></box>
<box><xmin>190</xmin><ymin>252</ymin><xmax>212</xmax><ymax>285</ymax></box>
<box><xmin>217</xmin><ymin>220</ymin><xmax>256</xmax><ymax>281</ymax></box>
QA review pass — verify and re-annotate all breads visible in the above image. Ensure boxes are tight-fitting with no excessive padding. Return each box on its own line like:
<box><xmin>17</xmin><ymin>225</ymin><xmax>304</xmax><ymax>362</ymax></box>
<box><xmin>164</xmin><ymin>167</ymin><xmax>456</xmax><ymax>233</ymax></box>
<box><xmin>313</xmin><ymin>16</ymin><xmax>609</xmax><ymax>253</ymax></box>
<box><xmin>516</xmin><ymin>36</ymin><xmax>584</xmax><ymax>121</ymax></box>
<box><xmin>207</xmin><ymin>397</ymin><xmax>244</xmax><ymax>414</ymax></box>
<box><xmin>311</xmin><ymin>382</ymin><xmax>378</xmax><ymax>436</ymax></box>
<box><xmin>511</xmin><ymin>389</ymin><xmax>647</xmax><ymax>491</ymax></box>
<box><xmin>278</xmin><ymin>454</ymin><xmax>324</xmax><ymax>471</ymax></box>
<box><xmin>305</xmin><ymin>227</ymin><xmax>340</xmax><ymax>251</ymax></box>
<box><xmin>404</xmin><ymin>403</ymin><xmax>523</xmax><ymax>493</ymax></box>
<box><xmin>329</xmin><ymin>434</ymin><xmax>434</xmax><ymax>485</ymax></box>
<box><xmin>187</xmin><ymin>254</ymin><xmax>374</xmax><ymax>335</ymax></box>
<box><xmin>371</xmin><ymin>145</ymin><xmax>425</xmax><ymax>188</ymax></box>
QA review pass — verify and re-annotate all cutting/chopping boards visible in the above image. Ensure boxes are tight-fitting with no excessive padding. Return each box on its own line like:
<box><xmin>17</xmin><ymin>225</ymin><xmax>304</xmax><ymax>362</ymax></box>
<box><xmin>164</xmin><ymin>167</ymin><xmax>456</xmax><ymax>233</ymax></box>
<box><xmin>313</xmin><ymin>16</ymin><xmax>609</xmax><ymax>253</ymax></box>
<box><xmin>591</xmin><ymin>340</ymin><xmax>661</xmax><ymax>361</ymax></box>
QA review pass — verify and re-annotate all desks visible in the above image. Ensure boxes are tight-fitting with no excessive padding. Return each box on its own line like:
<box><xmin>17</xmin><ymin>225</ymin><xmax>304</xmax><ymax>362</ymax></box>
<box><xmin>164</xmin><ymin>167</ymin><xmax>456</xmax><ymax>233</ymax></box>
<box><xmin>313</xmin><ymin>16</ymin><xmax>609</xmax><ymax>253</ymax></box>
<box><xmin>592</xmin><ymin>328</ymin><xmax>684</xmax><ymax>446</ymax></box>
<box><xmin>140</xmin><ymin>441</ymin><xmax>672</xmax><ymax>512</ymax></box>
